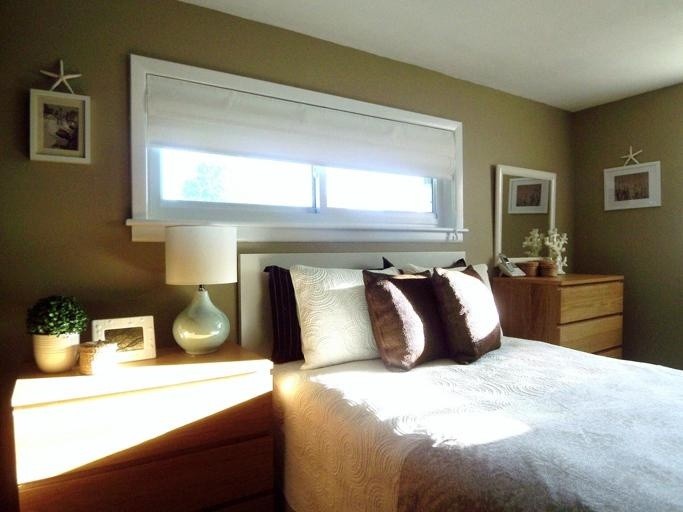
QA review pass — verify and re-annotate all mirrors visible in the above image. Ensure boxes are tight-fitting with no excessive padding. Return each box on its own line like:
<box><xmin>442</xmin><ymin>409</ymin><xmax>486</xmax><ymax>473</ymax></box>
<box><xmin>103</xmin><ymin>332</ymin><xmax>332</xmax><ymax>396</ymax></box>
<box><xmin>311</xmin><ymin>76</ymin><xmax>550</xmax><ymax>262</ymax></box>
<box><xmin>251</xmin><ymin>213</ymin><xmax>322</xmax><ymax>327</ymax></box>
<box><xmin>127</xmin><ymin>51</ymin><xmax>473</xmax><ymax>246</ymax></box>
<box><xmin>492</xmin><ymin>160</ymin><xmax>559</xmax><ymax>269</ymax></box>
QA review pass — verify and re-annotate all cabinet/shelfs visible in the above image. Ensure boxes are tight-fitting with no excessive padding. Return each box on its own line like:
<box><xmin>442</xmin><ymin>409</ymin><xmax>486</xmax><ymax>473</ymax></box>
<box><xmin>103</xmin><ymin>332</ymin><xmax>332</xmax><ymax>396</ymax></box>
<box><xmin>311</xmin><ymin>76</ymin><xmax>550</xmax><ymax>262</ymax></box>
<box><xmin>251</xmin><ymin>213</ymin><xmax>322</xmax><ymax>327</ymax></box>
<box><xmin>489</xmin><ymin>272</ymin><xmax>626</xmax><ymax>362</ymax></box>
<box><xmin>6</xmin><ymin>341</ymin><xmax>277</xmax><ymax>512</ymax></box>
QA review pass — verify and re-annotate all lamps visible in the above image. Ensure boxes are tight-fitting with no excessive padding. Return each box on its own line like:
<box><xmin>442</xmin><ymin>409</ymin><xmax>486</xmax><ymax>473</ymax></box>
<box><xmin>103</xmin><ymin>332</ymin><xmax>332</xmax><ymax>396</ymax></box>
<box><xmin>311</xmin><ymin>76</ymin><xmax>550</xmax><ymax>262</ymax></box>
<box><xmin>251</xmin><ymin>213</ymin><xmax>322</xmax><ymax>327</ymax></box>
<box><xmin>161</xmin><ymin>222</ymin><xmax>239</xmax><ymax>356</ymax></box>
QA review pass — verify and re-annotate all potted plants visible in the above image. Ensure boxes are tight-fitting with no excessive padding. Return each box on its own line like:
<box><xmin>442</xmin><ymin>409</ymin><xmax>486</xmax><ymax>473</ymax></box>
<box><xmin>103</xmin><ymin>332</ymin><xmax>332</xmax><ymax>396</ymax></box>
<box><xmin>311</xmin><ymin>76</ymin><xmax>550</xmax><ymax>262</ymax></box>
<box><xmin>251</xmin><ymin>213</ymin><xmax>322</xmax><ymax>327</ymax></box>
<box><xmin>24</xmin><ymin>290</ymin><xmax>87</xmax><ymax>376</ymax></box>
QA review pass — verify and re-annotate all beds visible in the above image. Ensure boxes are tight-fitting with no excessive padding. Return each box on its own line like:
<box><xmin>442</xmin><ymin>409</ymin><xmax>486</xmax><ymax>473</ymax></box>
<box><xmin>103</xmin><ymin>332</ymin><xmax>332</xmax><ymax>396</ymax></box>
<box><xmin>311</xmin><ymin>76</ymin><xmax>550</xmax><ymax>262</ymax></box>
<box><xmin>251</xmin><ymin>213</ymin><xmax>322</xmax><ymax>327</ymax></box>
<box><xmin>237</xmin><ymin>248</ymin><xmax>683</xmax><ymax>509</ymax></box>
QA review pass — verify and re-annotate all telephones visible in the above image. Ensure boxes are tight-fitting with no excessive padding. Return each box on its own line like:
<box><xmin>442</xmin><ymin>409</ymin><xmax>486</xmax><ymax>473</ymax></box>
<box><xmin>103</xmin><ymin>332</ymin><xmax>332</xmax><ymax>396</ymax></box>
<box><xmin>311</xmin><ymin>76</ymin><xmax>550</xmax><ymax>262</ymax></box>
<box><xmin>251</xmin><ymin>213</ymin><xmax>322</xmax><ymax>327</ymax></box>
<box><xmin>498</xmin><ymin>253</ymin><xmax>527</xmax><ymax>278</ymax></box>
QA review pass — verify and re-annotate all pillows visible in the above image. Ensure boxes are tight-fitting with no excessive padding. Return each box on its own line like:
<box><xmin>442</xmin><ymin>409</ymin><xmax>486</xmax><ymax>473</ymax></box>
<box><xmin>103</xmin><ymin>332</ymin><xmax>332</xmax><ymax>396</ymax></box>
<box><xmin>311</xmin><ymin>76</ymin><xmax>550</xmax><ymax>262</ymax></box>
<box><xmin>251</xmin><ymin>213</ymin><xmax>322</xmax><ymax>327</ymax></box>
<box><xmin>261</xmin><ymin>255</ymin><xmax>505</xmax><ymax>372</ymax></box>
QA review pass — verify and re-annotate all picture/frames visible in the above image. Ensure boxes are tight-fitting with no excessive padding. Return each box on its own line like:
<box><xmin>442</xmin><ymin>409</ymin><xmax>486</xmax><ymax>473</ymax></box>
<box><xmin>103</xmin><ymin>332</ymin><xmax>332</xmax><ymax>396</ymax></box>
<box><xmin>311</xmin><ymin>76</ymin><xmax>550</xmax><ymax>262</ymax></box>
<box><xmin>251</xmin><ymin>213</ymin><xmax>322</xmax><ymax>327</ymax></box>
<box><xmin>507</xmin><ymin>177</ymin><xmax>548</xmax><ymax>214</ymax></box>
<box><xmin>90</xmin><ymin>314</ymin><xmax>158</xmax><ymax>364</ymax></box>
<box><xmin>602</xmin><ymin>160</ymin><xmax>661</xmax><ymax>213</ymax></box>
<box><xmin>25</xmin><ymin>86</ymin><xmax>92</xmax><ymax>167</ymax></box>
